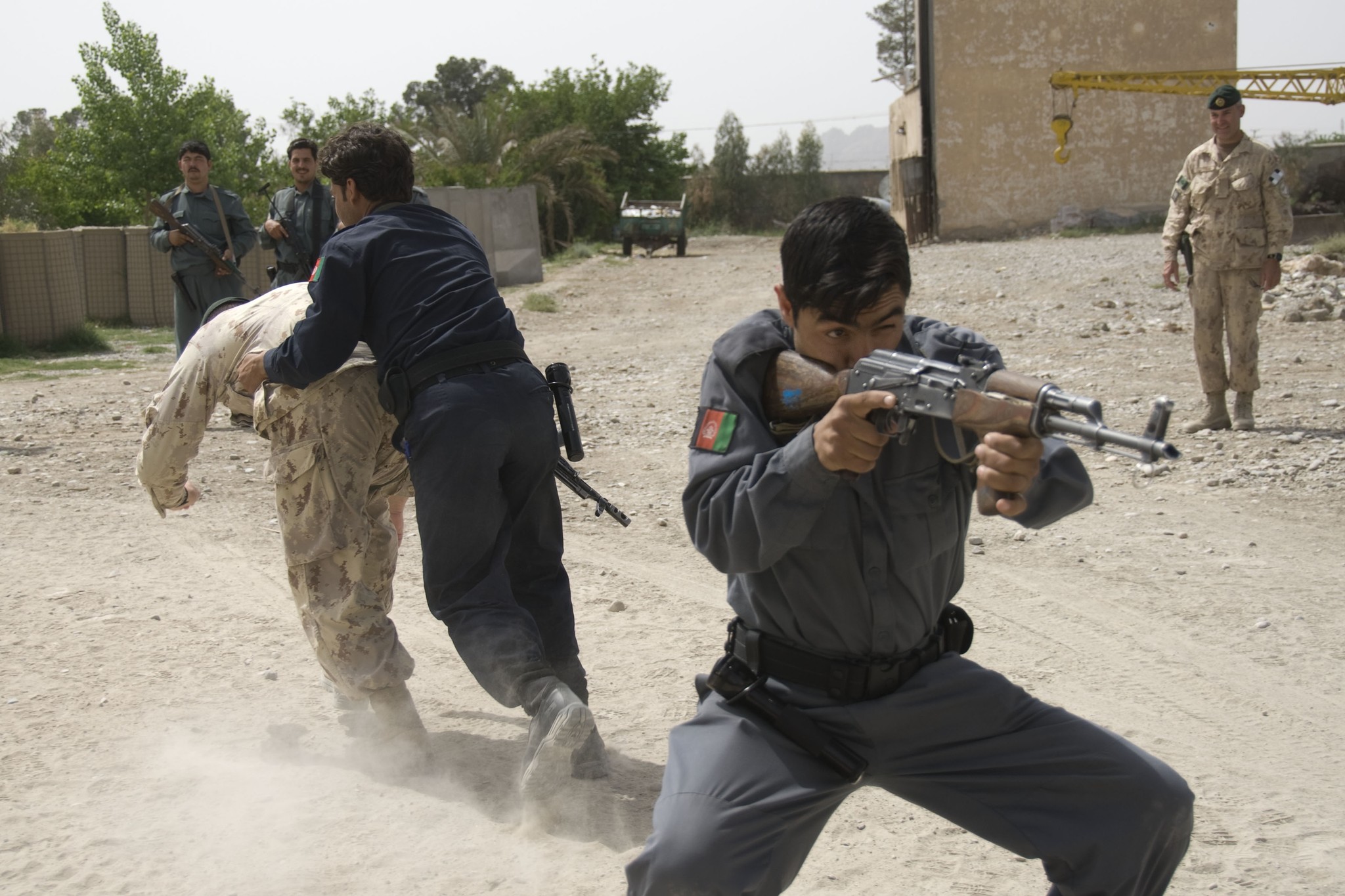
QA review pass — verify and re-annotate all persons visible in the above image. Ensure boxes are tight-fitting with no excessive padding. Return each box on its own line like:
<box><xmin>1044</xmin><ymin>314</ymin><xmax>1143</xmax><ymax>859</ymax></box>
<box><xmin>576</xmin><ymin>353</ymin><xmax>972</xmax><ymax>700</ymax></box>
<box><xmin>1162</xmin><ymin>85</ymin><xmax>1294</xmax><ymax>432</ymax></box>
<box><xmin>623</xmin><ymin>198</ymin><xmax>1196</xmax><ymax>896</ymax></box>
<box><xmin>137</xmin><ymin>128</ymin><xmax>606</xmax><ymax>805</ymax></box>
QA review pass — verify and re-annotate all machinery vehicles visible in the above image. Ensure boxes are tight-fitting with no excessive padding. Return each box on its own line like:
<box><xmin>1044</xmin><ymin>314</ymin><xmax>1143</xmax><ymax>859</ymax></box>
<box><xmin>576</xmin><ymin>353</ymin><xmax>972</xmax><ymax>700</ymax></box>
<box><xmin>1048</xmin><ymin>61</ymin><xmax>1345</xmax><ymax>165</ymax></box>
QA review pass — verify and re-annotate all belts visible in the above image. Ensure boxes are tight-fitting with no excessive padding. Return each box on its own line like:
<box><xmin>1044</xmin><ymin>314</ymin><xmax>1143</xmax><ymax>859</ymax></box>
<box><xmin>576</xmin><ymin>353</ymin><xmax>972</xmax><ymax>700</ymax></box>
<box><xmin>727</xmin><ymin>618</ymin><xmax>951</xmax><ymax>698</ymax></box>
<box><xmin>388</xmin><ymin>341</ymin><xmax>532</xmax><ymax>398</ymax></box>
<box><xmin>277</xmin><ymin>261</ymin><xmax>312</xmax><ymax>274</ymax></box>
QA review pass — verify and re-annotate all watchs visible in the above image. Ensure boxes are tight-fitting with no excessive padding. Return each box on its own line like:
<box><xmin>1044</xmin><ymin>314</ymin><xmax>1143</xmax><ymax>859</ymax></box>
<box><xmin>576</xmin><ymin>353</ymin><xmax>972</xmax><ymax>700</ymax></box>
<box><xmin>1268</xmin><ymin>253</ymin><xmax>1283</xmax><ymax>261</ymax></box>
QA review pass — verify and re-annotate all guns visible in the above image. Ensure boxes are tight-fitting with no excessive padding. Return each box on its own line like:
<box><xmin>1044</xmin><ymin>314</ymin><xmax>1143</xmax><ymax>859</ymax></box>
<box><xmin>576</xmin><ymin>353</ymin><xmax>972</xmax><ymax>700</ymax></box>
<box><xmin>149</xmin><ymin>199</ymin><xmax>262</xmax><ymax>298</ymax></box>
<box><xmin>762</xmin><ymin>349</ymin><xmax>1181</xmax><ymax>516</ymax></box>
<box><xmin>1181</xmin><ymin>234</ymin><xmax>1193</xmax><ymax>274</ymax></box>
<box><xmin>553</xmin><ymin>454</ymin><xmax>632</xmax><ymax>528</ymax></box>
<box><xmin>257</xmin><ymin>182</ymin><xmax>314</xmax><ymax>275</ymax></box>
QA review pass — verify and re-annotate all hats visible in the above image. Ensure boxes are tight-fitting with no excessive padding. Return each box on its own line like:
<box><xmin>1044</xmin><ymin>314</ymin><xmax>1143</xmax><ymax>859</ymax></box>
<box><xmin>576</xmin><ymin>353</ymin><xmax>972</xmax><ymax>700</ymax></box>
<box><xmin>1207</xmin><ymin>84</ymin><xmax>1241</xmax><ymax>110</ymax></box>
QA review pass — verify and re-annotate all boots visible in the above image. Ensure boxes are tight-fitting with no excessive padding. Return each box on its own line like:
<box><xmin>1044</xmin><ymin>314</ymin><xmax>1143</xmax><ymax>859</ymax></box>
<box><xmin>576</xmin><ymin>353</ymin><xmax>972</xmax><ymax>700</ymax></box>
<box><xmin>332</xmin><ymin>686</ymin><xmax>367</xmax><ymax>711</ymax></box>
<box><xmin>354</xmin><ymin>683</ymin><xmax>437</xmax><ymax>785</ymax></box>
<box><xmin>518</xmin><ymin>677</ymin><xmax>594</xmax><ymax>801</ymax></box>
<box><xmin>1184</xmin><ymin>391</ymin><xmax>1231</xmax><ymax>433</ymax></box>
<box><xmin>571</xmin><ymin>728</ymin><xmax>608</xmax><ymax>781</ymax></box>
<box><xmin>1232</xmin><ymin>392</ymin><xmax>1254</xmax><ymax>430</ymax></box>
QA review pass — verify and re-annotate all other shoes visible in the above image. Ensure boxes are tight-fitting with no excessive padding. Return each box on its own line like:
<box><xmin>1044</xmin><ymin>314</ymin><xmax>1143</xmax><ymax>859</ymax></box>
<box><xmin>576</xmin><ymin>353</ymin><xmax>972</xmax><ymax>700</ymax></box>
<box><xmin>229</xmin><ymin>394</ymin><xmax>255</xmax><ymax>426</ymax></box>
